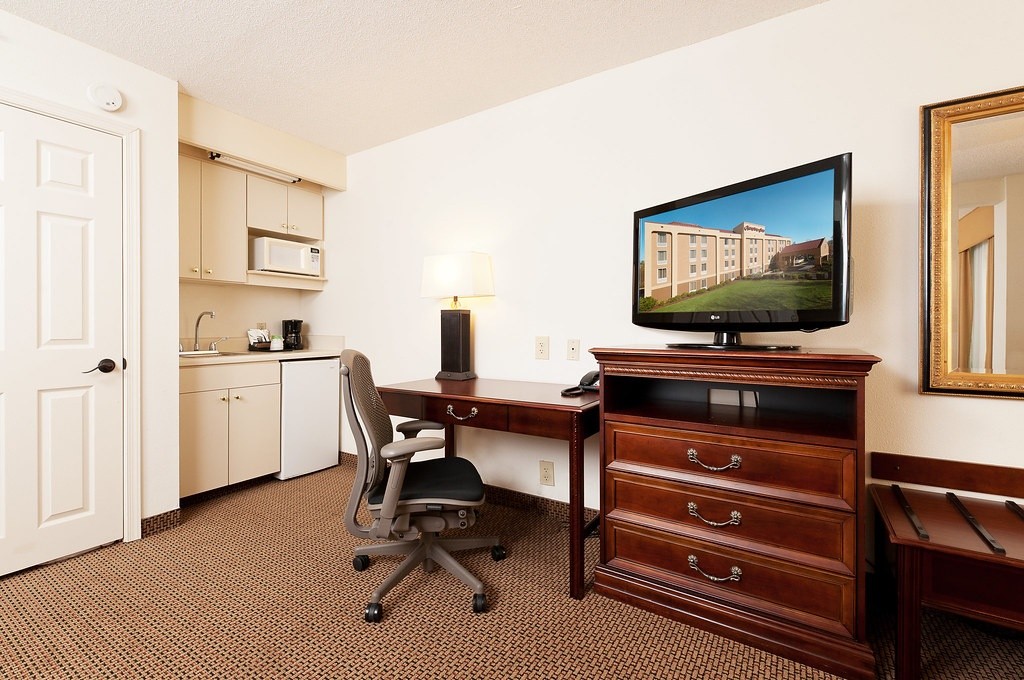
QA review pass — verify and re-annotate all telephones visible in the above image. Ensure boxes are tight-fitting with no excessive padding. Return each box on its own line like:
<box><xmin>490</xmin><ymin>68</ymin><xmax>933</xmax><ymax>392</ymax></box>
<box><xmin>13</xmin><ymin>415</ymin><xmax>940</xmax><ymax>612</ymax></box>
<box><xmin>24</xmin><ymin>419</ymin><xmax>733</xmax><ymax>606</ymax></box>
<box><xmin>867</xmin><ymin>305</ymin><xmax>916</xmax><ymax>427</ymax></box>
<box><xmin>577</xmin><ymin>371</ymin><xmax>600</xmax><ymax>390</ymax></box>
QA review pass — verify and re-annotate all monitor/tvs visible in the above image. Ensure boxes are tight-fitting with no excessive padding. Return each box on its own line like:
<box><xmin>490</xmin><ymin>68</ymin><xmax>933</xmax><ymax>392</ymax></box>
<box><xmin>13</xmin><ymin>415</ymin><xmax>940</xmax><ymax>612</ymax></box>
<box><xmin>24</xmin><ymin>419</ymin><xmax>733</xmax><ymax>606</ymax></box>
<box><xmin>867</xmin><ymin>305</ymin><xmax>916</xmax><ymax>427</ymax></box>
<box><xmin>631</xmin><ymin>153</ymin><xmax>854</xmax><ymax>349</ymax></box>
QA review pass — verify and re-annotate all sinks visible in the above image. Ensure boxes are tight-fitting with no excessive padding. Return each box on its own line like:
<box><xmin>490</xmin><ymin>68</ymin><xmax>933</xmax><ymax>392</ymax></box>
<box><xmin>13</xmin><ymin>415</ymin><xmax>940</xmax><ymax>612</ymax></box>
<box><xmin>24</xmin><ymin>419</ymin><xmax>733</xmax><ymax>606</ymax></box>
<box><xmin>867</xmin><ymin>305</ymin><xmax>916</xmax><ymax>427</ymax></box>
<box><xmin>179</xmin><ymin>351</ymin><xmax>250</xmax><ymax>358</ymax></box>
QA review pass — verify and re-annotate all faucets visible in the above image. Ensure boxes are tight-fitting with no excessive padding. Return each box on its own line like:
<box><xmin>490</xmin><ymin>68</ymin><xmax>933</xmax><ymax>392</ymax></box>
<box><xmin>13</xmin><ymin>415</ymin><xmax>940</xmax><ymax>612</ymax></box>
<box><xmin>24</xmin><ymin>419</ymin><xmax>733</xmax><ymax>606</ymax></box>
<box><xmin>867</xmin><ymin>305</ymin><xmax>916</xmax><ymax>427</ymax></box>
<box><xmin>194</xmin><ymin>312</ymin><xmax>215</xmax><ymax>351</ymax></box>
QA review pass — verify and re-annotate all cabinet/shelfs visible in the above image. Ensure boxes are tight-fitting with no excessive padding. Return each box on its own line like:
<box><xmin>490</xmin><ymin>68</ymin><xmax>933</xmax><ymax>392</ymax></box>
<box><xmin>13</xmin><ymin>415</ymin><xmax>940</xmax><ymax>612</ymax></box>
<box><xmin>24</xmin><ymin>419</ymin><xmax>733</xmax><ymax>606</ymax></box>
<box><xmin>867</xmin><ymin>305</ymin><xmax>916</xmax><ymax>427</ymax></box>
<box><xmin>866</xmin><ymin>480</ymin><xmax>1024</xmax><ymax>680</ymax></box>
<box><xmin>588</xmin><ymin>347</ymin><xmax>882</xmax><ymax>680</ymax></box>
<box><xmin>178</xmin><ymin>153</ymin><xmax>246</xmax><ymax>284</ymax></box>
<box><xmin>180</xmin><ymin>361</ymin><xmax>282</xmax><ymax>497</ymax></box>
<box><xmin>247</xmin><ymin>174</ymin><xmax>323</xmax><ymax>240</ymax></box>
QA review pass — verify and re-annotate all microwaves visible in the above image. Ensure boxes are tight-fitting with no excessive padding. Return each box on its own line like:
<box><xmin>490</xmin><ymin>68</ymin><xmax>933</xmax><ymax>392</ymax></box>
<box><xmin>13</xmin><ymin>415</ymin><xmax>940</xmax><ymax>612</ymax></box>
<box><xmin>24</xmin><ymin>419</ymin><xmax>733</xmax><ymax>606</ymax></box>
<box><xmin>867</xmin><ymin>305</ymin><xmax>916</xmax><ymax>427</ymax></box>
<box><xmin>248</xmin><ymin>236</ymin><xmax>321</xmax><ymax>277</ymax></box>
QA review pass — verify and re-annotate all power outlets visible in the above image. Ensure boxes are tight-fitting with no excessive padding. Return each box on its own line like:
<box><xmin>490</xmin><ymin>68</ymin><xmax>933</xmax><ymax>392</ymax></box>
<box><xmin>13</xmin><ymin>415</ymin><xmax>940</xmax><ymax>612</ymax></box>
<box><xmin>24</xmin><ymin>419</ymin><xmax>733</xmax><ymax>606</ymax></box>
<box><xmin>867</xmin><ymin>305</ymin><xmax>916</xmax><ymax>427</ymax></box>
<box><xmin>534</xmin><ymin>336</ymin><xmax>550</xmax><ymax>360</ymax></box>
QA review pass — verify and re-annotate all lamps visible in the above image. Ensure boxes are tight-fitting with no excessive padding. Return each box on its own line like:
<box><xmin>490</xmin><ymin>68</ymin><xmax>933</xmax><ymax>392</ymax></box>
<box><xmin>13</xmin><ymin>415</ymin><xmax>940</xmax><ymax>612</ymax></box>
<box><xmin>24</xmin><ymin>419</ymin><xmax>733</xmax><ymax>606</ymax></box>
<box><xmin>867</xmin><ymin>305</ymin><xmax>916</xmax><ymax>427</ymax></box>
<box><xmin>430</xmin><ymin>253</ymin><xmax>494</xmax><ymax>383</ymax></box>
<box><xmin>208</xmin><ymin>151</ymin><xmax>302</xmax><ymax>183</ymax></box>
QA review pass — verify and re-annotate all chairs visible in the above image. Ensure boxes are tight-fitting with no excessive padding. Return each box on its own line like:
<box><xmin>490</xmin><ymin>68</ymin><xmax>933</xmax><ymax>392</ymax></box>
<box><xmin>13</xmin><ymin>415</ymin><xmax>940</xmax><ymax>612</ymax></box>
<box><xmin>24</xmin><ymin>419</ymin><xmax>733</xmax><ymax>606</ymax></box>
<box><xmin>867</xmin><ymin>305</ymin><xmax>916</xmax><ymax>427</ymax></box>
<box><xmin>375</xmin><ymin>377</ymin><xmax>601</xmax><ymax>601</ymax></box>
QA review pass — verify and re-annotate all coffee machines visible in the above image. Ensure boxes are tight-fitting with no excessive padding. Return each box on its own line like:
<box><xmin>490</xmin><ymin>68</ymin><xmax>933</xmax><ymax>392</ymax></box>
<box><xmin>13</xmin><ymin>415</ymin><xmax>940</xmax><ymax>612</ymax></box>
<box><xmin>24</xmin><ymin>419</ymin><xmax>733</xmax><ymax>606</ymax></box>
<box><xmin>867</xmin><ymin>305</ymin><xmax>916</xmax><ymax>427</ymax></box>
<box><xmin>282</xmin><ymin>320</ymin><xmax>303</xmax><ymax>350</ymax></box>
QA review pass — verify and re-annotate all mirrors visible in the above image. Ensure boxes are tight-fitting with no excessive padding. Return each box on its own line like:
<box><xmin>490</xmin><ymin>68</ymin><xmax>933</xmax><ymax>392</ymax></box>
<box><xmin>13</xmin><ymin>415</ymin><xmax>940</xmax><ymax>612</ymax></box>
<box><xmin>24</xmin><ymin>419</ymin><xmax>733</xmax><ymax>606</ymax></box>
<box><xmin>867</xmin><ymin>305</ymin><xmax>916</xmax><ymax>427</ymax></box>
<box><xmin>917</xmin><ymin>85</ymin><xmax>1024</xmax><ymax>401</ymax></box>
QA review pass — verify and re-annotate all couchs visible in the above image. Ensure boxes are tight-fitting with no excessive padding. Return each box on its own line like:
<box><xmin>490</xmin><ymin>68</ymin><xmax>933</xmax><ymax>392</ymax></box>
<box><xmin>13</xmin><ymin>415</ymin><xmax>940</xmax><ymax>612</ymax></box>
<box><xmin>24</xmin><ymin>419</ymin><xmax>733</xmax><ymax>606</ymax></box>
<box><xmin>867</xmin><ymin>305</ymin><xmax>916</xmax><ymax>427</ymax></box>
<box><xmin>338</xmin><ymin>349</ymin><xmax>507</xmax><ymax>624</ymax></box>
